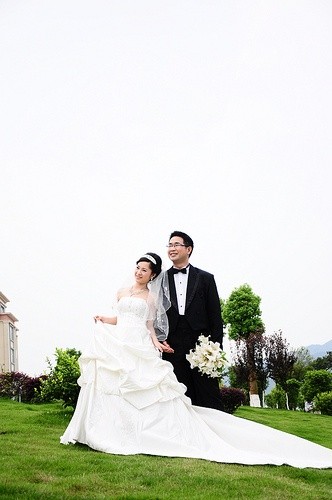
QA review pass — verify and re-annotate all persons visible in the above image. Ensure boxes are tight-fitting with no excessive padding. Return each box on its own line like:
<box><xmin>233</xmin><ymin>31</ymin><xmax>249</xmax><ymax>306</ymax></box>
<box><xmin>155</xmin><ymin>231</ymin><xmax>225</xmax><ymax>411</ymax></box>
<box><xmin>94</xmin><ymin>253</ymin><xmax>175</xmax><ymax>456</ymax></box>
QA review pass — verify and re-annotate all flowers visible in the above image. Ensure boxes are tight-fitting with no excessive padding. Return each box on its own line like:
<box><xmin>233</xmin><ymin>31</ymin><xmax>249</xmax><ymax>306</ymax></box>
<box><xmin>186</xmin><ymin>333</ymin><xmax>229</xmax><ymax>383</ymax></box>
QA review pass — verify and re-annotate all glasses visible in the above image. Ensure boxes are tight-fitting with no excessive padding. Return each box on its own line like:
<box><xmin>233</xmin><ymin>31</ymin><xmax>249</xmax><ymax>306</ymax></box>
<box><xmin>166</xmin><ymin>244</ymin><xmax>189</xmax><ymax>248</ymax></box>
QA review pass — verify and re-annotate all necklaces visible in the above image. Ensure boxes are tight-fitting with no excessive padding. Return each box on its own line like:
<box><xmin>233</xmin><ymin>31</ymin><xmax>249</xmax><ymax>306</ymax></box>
<box><xmin>129</xmin><ymin>286</ymin><xmax>149</xmax><ymax>296</ymax></box>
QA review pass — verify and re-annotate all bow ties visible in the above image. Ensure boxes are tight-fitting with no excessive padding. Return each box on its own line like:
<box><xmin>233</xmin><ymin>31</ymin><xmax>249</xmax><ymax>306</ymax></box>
<box><xmin>171</xmin><ymin>263</ymin><xmax>190</xmax><ymax>274</ymax></box>
